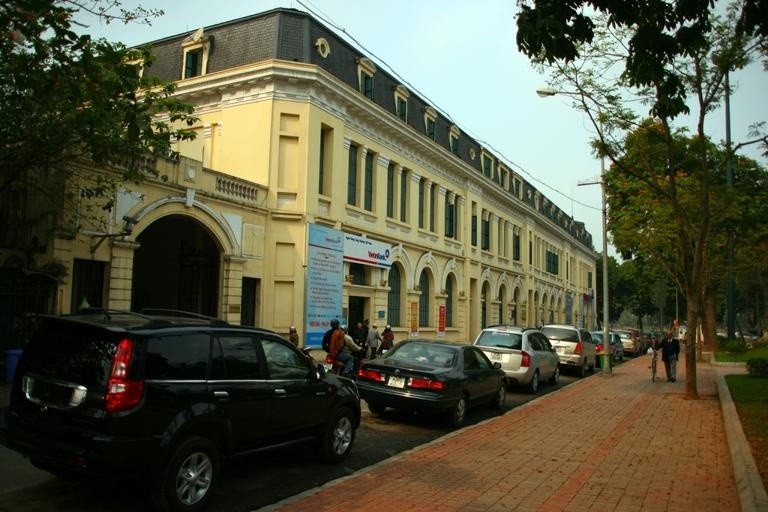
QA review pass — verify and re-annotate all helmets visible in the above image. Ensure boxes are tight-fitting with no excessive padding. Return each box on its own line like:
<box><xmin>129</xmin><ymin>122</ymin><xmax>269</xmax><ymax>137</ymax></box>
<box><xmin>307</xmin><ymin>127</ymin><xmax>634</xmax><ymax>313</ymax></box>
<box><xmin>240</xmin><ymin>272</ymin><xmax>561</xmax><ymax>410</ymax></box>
<box><xmin>341</xmin><ymin>324</ymin><xmax>348</xmax><ymax>333</ymax></box>
<box><xmin>330</xmin><ymin>319</ymin><xmax>338</xmax><ymax>328</ymax></box>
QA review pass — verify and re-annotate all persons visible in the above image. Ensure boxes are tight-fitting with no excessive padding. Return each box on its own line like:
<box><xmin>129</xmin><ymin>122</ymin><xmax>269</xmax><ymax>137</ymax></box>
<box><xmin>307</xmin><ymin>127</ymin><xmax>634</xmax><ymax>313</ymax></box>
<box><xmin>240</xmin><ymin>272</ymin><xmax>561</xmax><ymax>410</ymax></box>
<box><xmin>321</xmin><ymin>319</ymin><xmax>342</xmax><ymax>354</ymax></box>
<box><xmin>352</xmin><ymin>322</ymin><xmax>362</xmax><ymax>341</ymax></box>
<box><xmin>655</xmin><ymin>329</ymin><xmax>680</xmax><ymax>383</ymax></box>
<box><xmin>365</xmin><ymin>323</ymin><xmax>384</xmax><ymax>359</ymax></box>
<box><xmin>330</xmin><ymin>325</ymin><xmax>364</xmax><ymax>376</ymax></box>
<box><xmin>376</xmin><ymin>324</ymin><xmax>394</xmax><ymax>355</ymax></box>
<box><xmin>358</xmin><ymin>319</ymin><xmax>370</xmax><ymax>358</ymax></box>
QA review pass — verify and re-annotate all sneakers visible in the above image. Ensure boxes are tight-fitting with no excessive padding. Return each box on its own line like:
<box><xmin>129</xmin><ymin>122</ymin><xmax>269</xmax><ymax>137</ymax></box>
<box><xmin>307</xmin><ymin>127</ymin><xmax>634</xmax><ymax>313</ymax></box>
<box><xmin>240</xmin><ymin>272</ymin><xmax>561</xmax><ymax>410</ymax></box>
<box><xmin>667</xmin><ymin>378</ymin><xmax>676</xmax><ymax>383</ymax></box>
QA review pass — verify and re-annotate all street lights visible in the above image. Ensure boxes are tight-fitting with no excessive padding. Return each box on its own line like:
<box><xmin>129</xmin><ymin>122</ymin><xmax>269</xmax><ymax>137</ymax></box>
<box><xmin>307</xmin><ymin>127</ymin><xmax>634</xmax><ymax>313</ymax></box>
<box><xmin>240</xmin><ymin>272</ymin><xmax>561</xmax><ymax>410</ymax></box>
<box><xmin>537</xmin><ymin>88</ymin><xmax>612</xmax><ymax>377</ymax></box>
<box><xmin>712</xmin><ymin>50</ymin><xmax>737</xmax><ymax>338</ymax></box>
